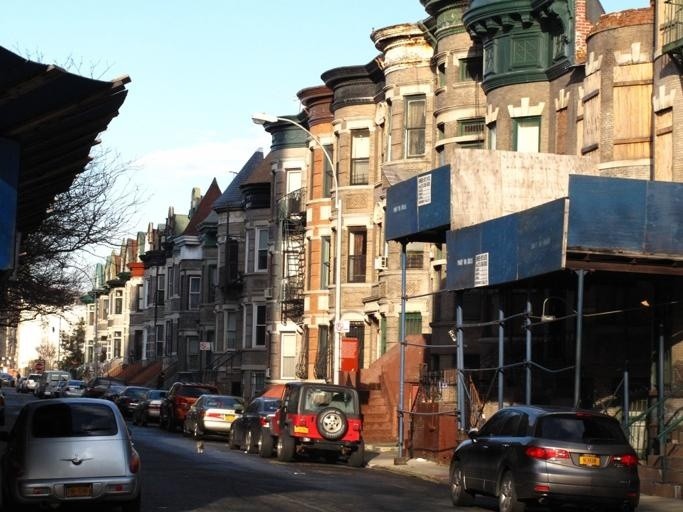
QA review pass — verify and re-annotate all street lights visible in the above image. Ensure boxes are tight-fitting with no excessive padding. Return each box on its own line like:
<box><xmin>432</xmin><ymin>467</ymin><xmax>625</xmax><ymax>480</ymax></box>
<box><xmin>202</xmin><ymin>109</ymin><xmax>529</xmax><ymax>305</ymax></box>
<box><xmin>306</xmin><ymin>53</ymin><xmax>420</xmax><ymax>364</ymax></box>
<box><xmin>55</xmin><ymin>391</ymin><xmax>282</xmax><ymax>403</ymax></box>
<box><xmin>57</xmin><ymin>261</ymin><xmax>98</xmax><ymax>376</ymax></box>
<box><xmin>246</xmin><ymin>107</ymin><xmax>342</xmax><ymax>388</ymax></box>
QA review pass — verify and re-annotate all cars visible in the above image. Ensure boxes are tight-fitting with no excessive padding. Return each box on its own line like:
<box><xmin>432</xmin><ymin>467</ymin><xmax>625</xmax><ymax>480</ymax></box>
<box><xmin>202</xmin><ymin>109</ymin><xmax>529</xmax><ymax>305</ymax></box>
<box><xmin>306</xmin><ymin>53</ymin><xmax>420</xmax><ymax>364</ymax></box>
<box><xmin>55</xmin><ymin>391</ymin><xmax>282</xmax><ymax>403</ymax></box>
<box><xmin>444</xmin><ymin>404</ymin><xmax>643</xmax><ymax>512</ymax></box>
<box><xmin>5</xmin><ymin>396</ymin><xmax>143</xmax><ymax>512</ymax></box>
<box><xmin>224</xmin><ymin>394</ymin><xmax>279</xmax><ymax>455</ymax></box>
<box><xmin>0</xmin><ymin>368</ymin><xmax>243</xmax><ymax>440</ymax></box>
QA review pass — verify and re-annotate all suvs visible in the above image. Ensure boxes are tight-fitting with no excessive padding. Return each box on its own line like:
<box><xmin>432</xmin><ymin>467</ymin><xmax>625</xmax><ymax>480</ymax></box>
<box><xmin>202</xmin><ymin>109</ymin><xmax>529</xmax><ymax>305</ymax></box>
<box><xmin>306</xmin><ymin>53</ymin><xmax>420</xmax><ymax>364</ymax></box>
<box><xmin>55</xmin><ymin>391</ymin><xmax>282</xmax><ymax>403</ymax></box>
<box><xmin>254</xmin><ymin>381</ymin><xmax>366</xmax><ymax>469</ymax></box>
